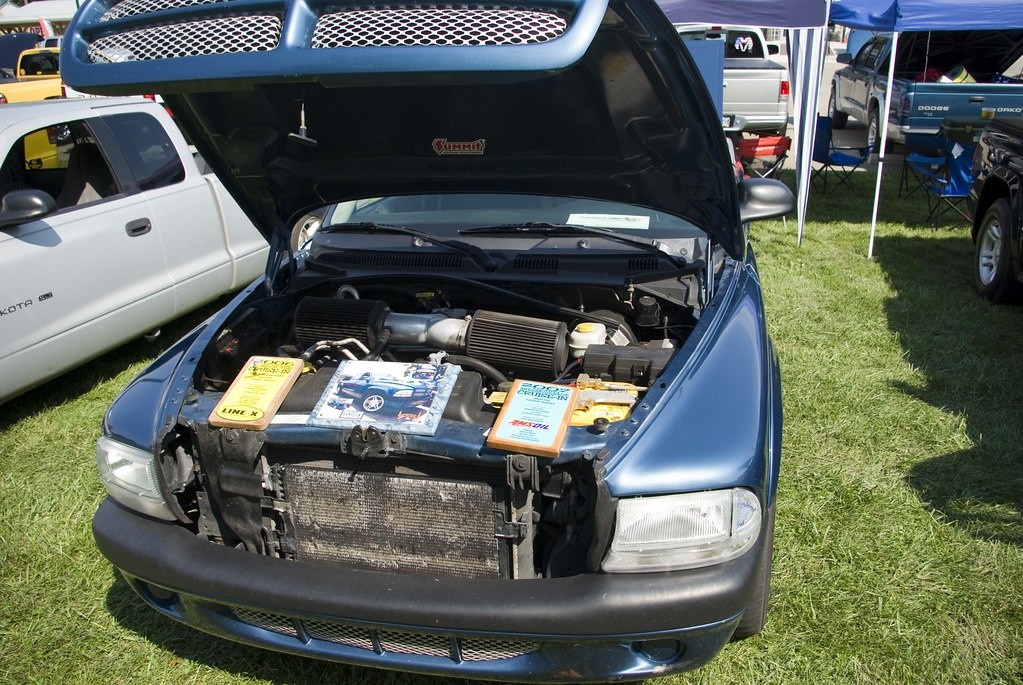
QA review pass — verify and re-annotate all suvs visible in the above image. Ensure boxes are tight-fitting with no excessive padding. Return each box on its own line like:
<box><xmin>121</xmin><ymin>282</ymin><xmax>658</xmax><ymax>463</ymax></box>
<box><xmin>40</xmin><ymin>37</ymin><xmax>66</xmax><ymax>48</ymax></box>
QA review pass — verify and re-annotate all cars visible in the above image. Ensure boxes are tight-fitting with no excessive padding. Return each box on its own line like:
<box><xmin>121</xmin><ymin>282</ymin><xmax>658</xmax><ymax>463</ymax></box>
<box><xmin>61</xmin><ymin>0</ymin><xmax>797</xmax><ymax>681</ymax></box>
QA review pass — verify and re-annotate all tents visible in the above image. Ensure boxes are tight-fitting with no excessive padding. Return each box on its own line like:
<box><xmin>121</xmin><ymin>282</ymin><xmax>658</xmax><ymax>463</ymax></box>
<box><xmin>655</xmin><ymin>0</ymin><xmax>1023</xmax><ymax>259</ymax></box>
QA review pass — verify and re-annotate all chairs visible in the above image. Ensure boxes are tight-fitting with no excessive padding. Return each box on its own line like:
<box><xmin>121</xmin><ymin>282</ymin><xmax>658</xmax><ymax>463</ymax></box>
<box><xmin>899</xmin><ymin>126</ymin><xmax>976</xmax><ymax>234</ymax></box>
<box><xmin>812</xmin><ymin>112</ymin><xmax>875</xmax><ymax>195</ymax></box>
<box><xmin>735</xmin><ymin>136</ymin><xmax>793</xmax><ymax>228</ymax></box>
<box><xmin>56</xmin><ymin>142</ymin><xmax>116</xmax><ymax>211</ymax></box>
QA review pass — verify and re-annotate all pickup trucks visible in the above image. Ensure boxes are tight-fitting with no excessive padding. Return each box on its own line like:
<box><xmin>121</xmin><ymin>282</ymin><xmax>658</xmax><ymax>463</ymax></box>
<box><xmin>1</xmin><ymin>98</ymin><xmax>272</xmax><ymax>403</ymax></box>
<box><xmin>0</xmin><ymin>46</ymin><xmax>63</xmax><ymax>106</ymax></box>
<box><xmin>674</xmin><ymin>21</ymin><xmax>789</xmax><ymax>135</ymax></box>
<box><xmin>966</xmin><ymin>122</ymin><xmax>1023</xmax><ymax>300</ymax></box>
<box><xmin>828</xmin><ymin>35</ymin><xmax>1023</xmax><ymax>152</ymax></box>
<box><xmin>63</xmin><ymin>81</ymin><xmax>173</xmax><ymax>121</ymax></box>
